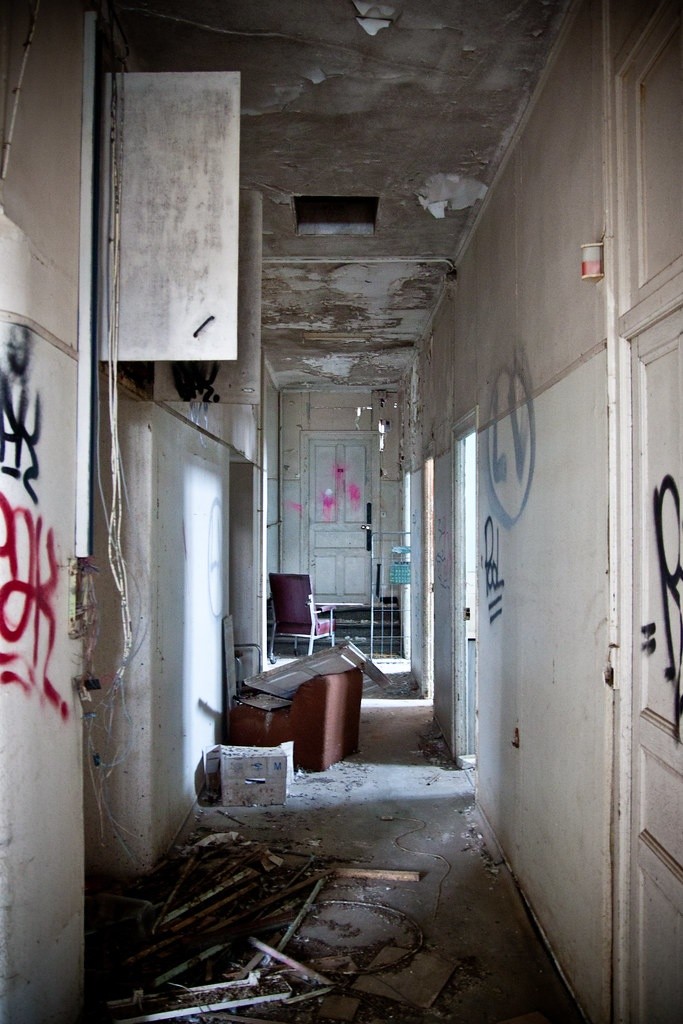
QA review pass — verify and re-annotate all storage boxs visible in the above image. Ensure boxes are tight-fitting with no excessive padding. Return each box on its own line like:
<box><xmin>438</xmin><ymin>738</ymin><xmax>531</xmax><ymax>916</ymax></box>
<box><xmin>201</xmin><ymin>740</ymin><xmax>295</xmax><ymax>807</ymax></box>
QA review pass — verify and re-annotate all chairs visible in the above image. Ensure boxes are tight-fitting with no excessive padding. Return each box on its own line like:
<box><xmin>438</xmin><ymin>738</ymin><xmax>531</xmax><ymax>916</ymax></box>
<box><xmin>269</xmin><ymin>572</ymin><xmax>337</xmax><ymax>664</ymax></box>
